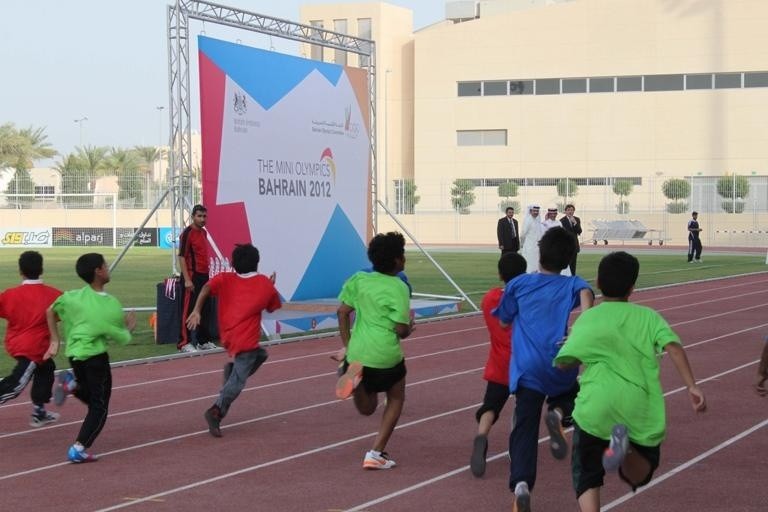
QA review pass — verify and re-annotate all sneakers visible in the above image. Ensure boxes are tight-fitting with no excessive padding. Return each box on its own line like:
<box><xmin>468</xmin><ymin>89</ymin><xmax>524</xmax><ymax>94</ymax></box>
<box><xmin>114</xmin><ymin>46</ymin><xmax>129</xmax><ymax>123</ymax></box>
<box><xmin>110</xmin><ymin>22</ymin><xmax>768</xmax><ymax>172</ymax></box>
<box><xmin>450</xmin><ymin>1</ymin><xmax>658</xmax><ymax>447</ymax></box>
<box><xmin>204</xmin><ymin>404</ymin><xmax>224</xmax><ymax>437</ymax></box>
<box><xmin>335</xmin><ymin>361</ymin><xmax>363</xmax><ymax>399</ymax></box>
<box><xmin>223</xmin><ymin>362</ymin><xmax>234</xmax><ymax>385</ymax></box>
<box><xmin>601</xmin><ymin>424</ymin><xmax>629</xmax><ymax>472</ymax></box>
<box><xmin>695</xmin><ymin>259</ymin><xmax>703</xmax><ymax>263</ymax></box>
<box><xmin>688</xmin><ymin>260</ymin><xmax>694</xmax><ymax>263</ymax></box>
<box><xmin>67</xmin><ymin>444</ymin><xmax>99</xmax><ymax>463</ymax></box>
<box><xmin>544</xmin><ymin>411</ymin><xmax>569</xmax><ymax>460</ymax></box>
<box><xmin>470</xmin><ymin>434</ymin><xmax>488</xmax><ymax>478</ymax></box>
<box><xmin>55</xmin><ymin>370</ymin><xmax>77</xmax><ymax>406</ymax></box>
<box><xmin>179</xmin><ymin>342</ymin><xmax>198</xmax><ymax>353</ymax></box>
<box><xmin>198</xmin><ymin>341</ymin><xmax>216</xmax><ymax>351</ymax></box>
<box><xmin>512</xmin><ymin>481</ymin><xmax>531</xmax><ymax>512</ymax></box>
<box><xmin>30</xmin><ymin>410</ymin><xmax>60</xmax><ymax>427</ymax></box>
<box><xmin>362</xmin><ymin>451</ymin><xmax>397</xmax><ymax>470</ymax></box>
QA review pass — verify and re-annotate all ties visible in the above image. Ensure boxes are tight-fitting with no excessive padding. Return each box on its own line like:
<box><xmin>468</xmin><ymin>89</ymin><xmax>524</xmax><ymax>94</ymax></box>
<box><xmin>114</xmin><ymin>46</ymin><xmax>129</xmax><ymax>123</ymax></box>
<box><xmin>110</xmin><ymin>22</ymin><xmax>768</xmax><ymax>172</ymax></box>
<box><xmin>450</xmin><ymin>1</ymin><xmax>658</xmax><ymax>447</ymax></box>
<box><xmin>510</xmin><ymin>220</ymin><xmax>514</xmax><ymax>239</ymax></box>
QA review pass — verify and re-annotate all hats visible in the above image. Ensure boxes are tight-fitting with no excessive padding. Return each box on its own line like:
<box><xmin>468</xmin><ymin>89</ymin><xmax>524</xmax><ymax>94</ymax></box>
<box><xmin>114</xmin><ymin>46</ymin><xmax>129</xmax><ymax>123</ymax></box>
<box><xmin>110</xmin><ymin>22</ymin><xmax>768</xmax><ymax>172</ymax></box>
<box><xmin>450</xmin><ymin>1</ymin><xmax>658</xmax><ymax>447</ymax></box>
<box><xmin>531</xmin><ymin>207</ymin><xmax>540</xmax><ymax>210</ymax></box>
<box><xmin>548</xmin><ymin>209</ymin><xmax>557</xmax><ymax>213</ymax></box>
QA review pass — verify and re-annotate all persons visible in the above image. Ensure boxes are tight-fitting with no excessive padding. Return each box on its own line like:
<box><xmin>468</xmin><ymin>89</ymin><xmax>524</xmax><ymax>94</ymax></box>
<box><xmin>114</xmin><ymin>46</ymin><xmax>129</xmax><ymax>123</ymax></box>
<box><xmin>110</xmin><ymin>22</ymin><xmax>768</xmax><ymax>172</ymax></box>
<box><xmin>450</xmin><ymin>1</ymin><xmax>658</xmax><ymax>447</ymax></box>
<box><xmin>468</xmin><ymin>252</ymin><xmax>528</xmax><ymax>476</ymax></box>
<box><xmin>1</xmin><ymin>249</ymin><xmax>66</xmax><ymax>428</ymax></box>
<box><xmin>497</xmin><ymin>207</ymin><xmax>520</xmax><ymax>258</ymax></box>
<box><xmin>330</xmin><ymin>233</ymin><xmax>417</xmax><ymax>468</ymax></box>
<box><xmin>520</xmin><ymin>203</ymin><xmax>543</xmax><ymax>274</ymax></box>
<box><xmin>179</xmin><ymin>204</ymin><xmax>224</xmax><ymax>353</ymax></box>
<box><xmin>493</xmin><ymin>227</ymin><xmax>595</xmax><ymax>512</ymax></box>
<box><xmin>753</xmin><ymin>342</ymin><xmax>768</xmax><ymax>399</ymax></box>
<box><xmin>687</xmin><ymin>211</ymin><xmax>704</xmax><ymax>264</ymax></box>
<box><xmin>185</xmin><ymin>244</ymin><xmax>282</xmax><ymax>438</ymax></box>
<box><xmin>542</xmin><ymin>206</ymin><xmax>572</xmax><ymax>276</ymax></box>
<box><xmin>559</xmin><ymin>205</ymin><xmax>582</xmax><ymax>275</ymax></box>
<box><xmin>44</xmin><ymin>252</ymin><xmax>138</xmax><ymax>463</ymax></box>
<box><xmin>550</xmin><ymin>249</ymin><xmax>708</xmax><ymax>511</ymax></box>
